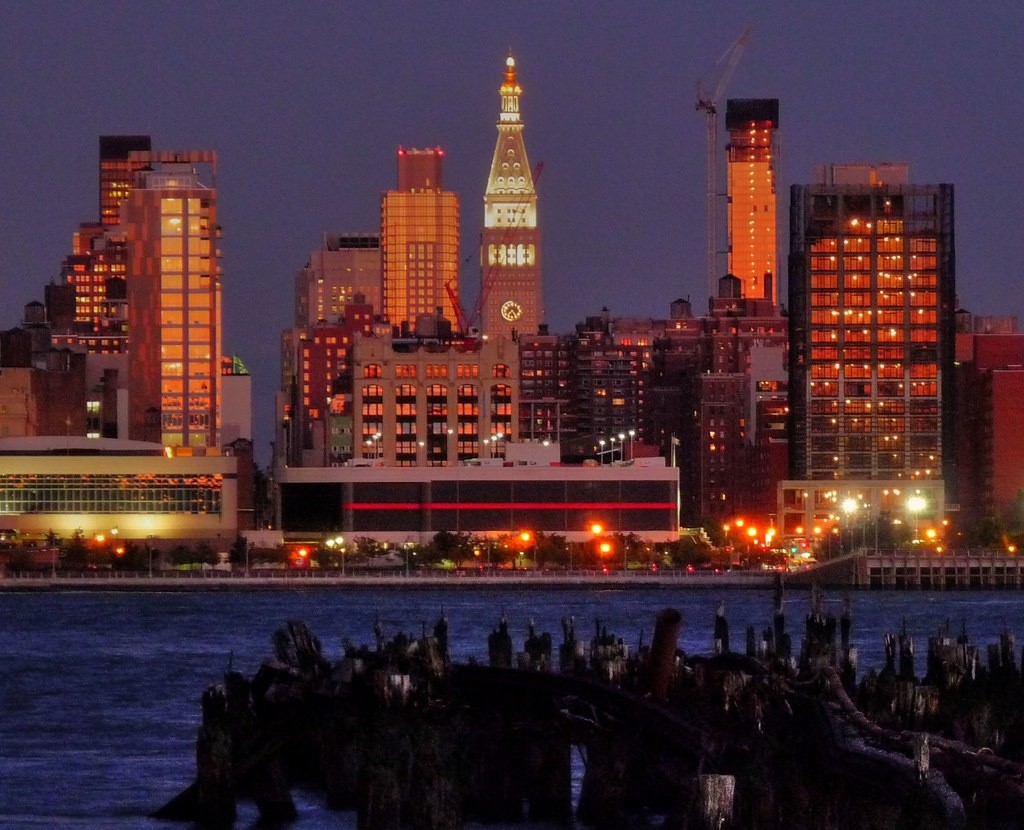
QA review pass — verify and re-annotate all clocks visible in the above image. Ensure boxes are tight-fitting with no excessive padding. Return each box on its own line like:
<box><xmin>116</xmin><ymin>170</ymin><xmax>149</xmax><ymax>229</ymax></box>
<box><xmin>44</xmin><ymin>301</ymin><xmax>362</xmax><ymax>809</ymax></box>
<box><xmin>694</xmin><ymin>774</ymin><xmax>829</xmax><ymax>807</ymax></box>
<box><xmin>500</xmin><ymin>300</ymin><xmax>524</xmax><ymax>322</ymax></box>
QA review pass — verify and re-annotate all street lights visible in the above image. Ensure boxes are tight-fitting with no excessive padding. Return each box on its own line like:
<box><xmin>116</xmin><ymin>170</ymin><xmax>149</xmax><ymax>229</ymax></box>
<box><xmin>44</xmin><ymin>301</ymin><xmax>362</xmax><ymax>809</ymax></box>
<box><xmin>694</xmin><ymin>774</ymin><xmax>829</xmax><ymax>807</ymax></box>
<box><xmin>599</xmin><ymin>427</ymin><xmax>635</xmax><ymax>465</ymax></box>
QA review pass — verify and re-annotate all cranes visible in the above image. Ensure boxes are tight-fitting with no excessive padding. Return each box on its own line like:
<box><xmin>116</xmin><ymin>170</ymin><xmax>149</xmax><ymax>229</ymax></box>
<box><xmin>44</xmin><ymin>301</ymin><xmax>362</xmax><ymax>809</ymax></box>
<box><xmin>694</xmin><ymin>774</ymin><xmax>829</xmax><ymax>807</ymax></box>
<box><xmin>444</xmin><ymin>160</ymin><xmax>545</xmax><ymax>353</ymax></box>
<box><xmin>693</xmin><ymin>23</ymin><xmax>755</xmax><ymax>297</ymax></box>
